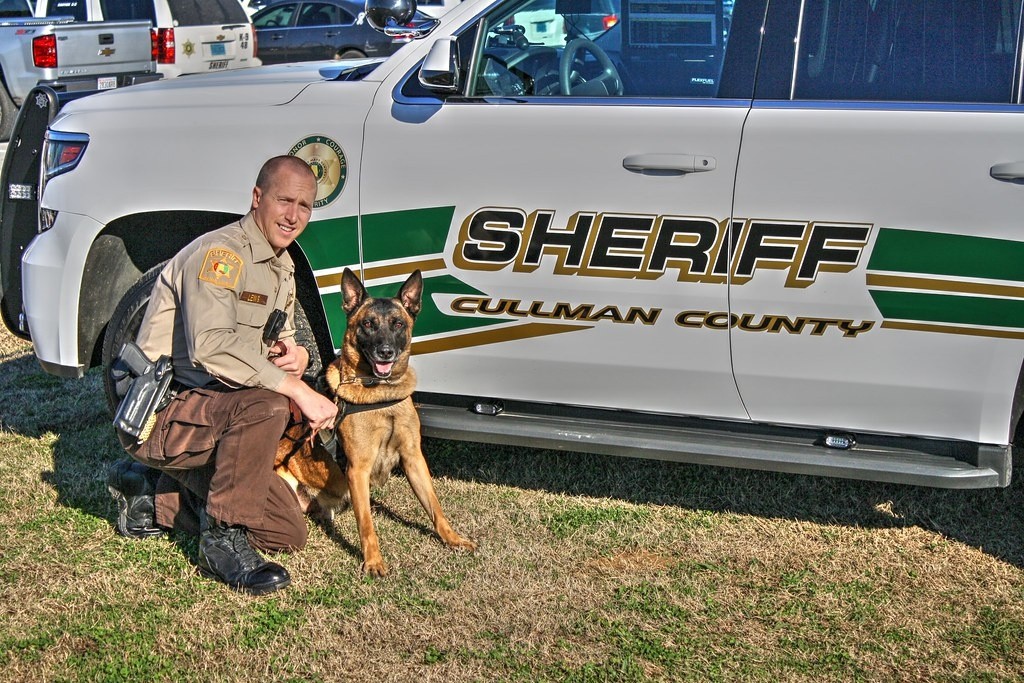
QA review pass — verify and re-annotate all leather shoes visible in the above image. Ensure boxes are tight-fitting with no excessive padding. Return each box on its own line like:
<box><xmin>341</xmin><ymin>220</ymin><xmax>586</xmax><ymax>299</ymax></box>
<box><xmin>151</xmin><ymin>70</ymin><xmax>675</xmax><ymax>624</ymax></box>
<box><xmin>107</xmin><ymin>458</ymin><xmax>164</xmax><ymax>540</ymax></box>
<box><xmin>198</xmin><ymin>507</ymin><xmax>291</xmax><ymax>593</ymax></box>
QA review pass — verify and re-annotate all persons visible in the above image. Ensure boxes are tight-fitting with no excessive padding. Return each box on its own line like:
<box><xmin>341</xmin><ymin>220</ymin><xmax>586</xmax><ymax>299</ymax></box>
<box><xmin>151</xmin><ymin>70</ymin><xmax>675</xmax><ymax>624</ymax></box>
<box><xmin>104</xmin><ymin>155</ymin><xmax>339</xmax><ymax>595</ymax></box>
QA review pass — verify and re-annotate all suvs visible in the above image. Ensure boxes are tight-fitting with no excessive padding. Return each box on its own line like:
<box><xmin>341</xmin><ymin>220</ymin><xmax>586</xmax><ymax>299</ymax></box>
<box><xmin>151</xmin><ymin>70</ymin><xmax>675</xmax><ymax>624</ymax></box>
<box><xmin>0</xmin><ymin>0</ymin><xmax>264</xmax><ymax>81</ymax></box>
<box><xmin>0</xmin><ymin>1</ymin><xmax>1023</xmax><ymax>504</ymax></box>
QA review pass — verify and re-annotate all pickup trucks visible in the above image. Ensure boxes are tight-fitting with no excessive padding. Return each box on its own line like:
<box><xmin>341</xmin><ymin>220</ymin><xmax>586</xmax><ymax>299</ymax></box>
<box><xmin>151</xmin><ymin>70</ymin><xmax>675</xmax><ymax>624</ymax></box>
<box><xmin>1</xmin><ymin>0</ymin><xmax>164</xmax><ymax>143</ymax></box>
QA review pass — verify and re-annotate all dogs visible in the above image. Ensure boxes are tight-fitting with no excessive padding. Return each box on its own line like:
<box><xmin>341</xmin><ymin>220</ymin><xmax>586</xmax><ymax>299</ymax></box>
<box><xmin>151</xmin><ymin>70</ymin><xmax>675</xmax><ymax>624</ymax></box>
<box><xmin>273</xmin><ymin>267</ymin><xmax>479</xmax><ymax>581</ymax></box>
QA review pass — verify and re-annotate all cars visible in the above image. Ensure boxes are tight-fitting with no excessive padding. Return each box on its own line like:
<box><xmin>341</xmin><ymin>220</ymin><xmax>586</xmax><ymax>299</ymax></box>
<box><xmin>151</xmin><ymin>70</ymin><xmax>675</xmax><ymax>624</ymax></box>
<box><xmin>249</xmin><ymin>3</ymin><xmax>440</xmax><ymax>66</ymax></box>
